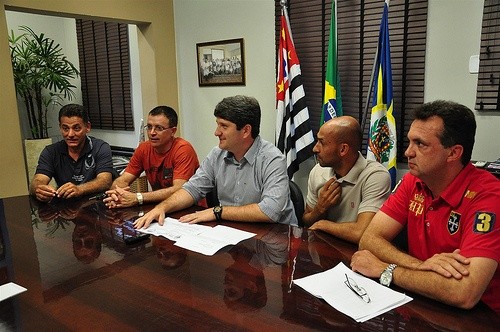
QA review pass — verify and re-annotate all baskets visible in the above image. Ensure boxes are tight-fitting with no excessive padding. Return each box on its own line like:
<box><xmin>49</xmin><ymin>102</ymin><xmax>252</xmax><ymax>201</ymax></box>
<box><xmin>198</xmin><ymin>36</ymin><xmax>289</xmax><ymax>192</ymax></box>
<box><xmin>129</xmin><ymin>176</ymin><xmax>148</xmax><ymax>194</ymax></box>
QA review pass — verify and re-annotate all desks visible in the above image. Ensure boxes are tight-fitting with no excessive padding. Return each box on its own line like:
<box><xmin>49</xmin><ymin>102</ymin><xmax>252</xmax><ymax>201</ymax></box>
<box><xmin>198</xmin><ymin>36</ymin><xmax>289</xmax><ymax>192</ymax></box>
<box><xmin>0</xmin><ymin>191</ymin><xmax>500</xmax><ymax>332</ymax></box>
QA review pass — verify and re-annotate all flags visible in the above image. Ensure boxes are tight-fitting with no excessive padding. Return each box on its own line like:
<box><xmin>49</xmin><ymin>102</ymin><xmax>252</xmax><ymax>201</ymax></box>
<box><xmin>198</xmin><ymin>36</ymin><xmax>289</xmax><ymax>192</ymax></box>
<box><xmin>366</xmin><ymin>2</ymin><xmax>398</xmax><ymax>192</ymax></box>
<box><xmin>320</xmin><ymin>0</ymin><xmax>344</xmax><ymax>128</ymax></box>
<box><xmin>275</xmin><ymin>9</ymin><xmax>315</xmax><ymax>183</ymax></box>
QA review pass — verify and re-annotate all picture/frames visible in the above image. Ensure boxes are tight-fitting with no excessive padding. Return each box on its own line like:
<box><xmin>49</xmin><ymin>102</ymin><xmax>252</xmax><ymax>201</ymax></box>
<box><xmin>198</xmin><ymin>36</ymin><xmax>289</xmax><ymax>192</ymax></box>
<box><xmin>196</xmin><ymin>38</ymin><xmax>245</xmax><ymax>87</ymax></box>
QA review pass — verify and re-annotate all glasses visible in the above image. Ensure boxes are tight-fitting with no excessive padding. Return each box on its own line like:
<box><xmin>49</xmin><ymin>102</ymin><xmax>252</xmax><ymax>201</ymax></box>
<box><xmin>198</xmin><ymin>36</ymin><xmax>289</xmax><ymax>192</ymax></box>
<box><xmin>144</xmin><ymin>125</ymin><xmax>172</xmax><ymax>132</ymax></box>
<box><xmin>344</xmin><ymin>274</ymin><xmax>371</xmax><ymax>303</ymax></box>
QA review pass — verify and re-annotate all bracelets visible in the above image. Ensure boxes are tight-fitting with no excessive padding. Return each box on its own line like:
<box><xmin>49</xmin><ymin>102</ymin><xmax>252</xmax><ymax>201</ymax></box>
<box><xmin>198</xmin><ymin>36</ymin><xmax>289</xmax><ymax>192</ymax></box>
<box><xmin>136</xmin><ymin>193</ymin><xmax>143</xmax><ymax>204</ymax></box>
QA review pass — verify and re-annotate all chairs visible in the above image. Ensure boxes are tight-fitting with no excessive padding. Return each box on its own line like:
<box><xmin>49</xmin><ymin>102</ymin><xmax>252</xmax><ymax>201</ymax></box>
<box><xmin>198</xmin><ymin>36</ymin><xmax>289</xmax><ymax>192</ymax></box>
<box><xmin>205</xmin><ymin>180</ymin><xmax>305</xmax><ymax>228</ymax></box>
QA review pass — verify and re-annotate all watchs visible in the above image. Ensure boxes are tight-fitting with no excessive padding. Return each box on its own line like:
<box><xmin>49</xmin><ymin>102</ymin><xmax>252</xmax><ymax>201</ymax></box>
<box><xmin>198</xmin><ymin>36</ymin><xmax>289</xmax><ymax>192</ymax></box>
<box><xmin>213</xmin><ymin>206</ymin><xmax>222</xmax><ymax>220</ymax></box>
<box><xmin>379</xmin><ymin>263</ymin><xmax>398</xmax><ymax>286</ymax></box>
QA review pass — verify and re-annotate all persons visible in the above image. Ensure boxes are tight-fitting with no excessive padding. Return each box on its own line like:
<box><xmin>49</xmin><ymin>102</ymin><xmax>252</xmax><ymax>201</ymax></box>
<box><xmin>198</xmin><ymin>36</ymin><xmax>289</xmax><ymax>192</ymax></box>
<box><xmin>218</xmin><ymin>225</ymin><xmax>289</xmax><ymax>314</ymax></box>
<box><xmin>151</xmin><ymin>236</ymin><xmax>186</xmax><ymax>270</ymax></box>
<box><xmin>133</xmin><ymin>95</ymin><xmax>298</xmax><ymax>229</ymax></box>
<box><xmin>30</xmin><ymin>104</ymin><xmax>113</xmax><ymax>203</ymax></box>
<box><xmin>303</xmin><ymin>115</ymin><xmax>392</xmax><ymax>244</ymax></box>
<box><xmin>37</xmin><ymin>203</ymin><xmax>103</xmax><ymax>265</ymax></box>
<box><xmin>350</xmin><ymin>101</ymin><xmax>500</xmax><ymax>309</ymax></box>
<box><xmin>201</xmin><ymin>56</ymin><xmax>242</xmax><ymax>82</ymax></box>
<box><xmin>103</xmin><ymin>106</ymin><xmax>207</xmax><ymax>209</ymax></box>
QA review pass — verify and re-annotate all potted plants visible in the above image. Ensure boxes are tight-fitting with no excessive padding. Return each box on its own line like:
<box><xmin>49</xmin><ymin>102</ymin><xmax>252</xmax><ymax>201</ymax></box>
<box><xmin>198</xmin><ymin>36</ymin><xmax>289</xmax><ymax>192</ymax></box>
<box><xmin>8</xmin><ymin>24</ymin><xmax>82</xmax><ymax>191</ymax></box>
